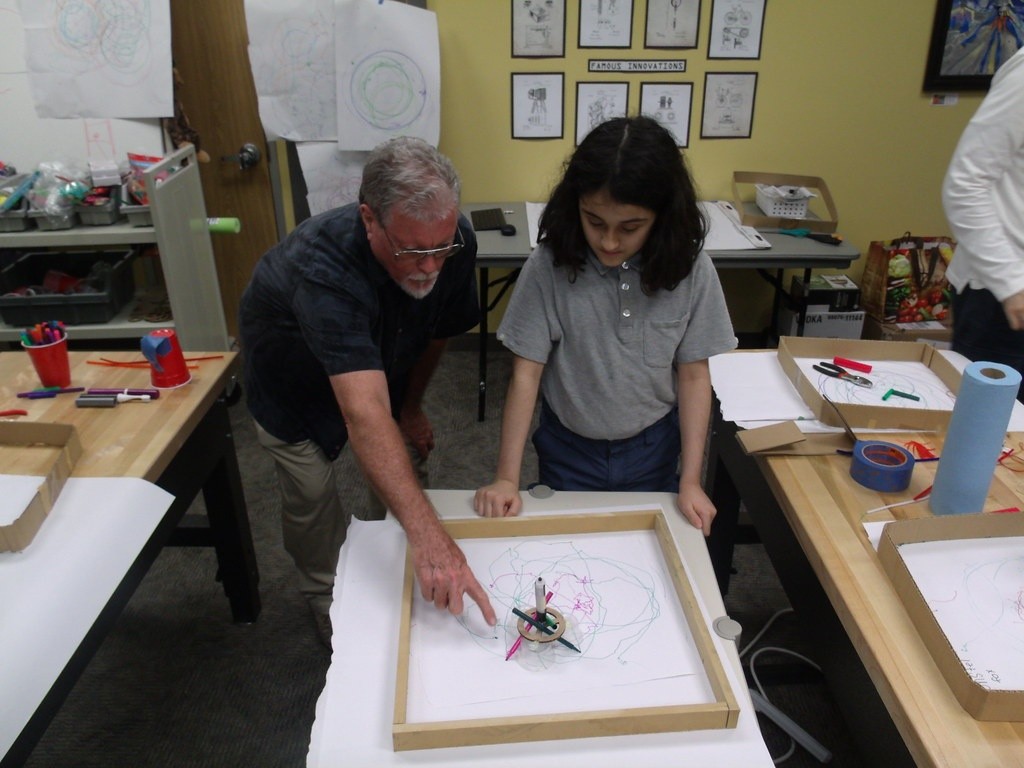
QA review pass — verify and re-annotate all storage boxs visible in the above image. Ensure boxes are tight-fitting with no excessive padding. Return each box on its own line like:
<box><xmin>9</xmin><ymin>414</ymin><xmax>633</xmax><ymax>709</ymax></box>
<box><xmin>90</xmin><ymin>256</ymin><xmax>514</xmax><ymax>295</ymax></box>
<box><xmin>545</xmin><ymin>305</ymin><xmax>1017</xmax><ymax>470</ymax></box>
<box><xmin>778</xmin><ymin>273</ymin><xmax>953</xmax><ymax>351</ymax></box>
<box><xmin>0</xmin><ymin>194</ymin><xmax>153</xmax><ymax>326</ymax></box>
<box><xmin>754</xmin><ymin>184</ymin><xmax>808</xmax><ymax>218</ymax></box>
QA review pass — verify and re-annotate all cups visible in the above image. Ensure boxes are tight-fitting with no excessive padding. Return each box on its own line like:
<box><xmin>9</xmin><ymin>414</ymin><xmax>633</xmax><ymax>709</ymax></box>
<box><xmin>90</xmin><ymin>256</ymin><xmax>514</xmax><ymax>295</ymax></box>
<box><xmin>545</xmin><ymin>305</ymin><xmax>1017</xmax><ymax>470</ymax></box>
<box><xmin>149</xmin><ymin>329</ymin><xmax>192</xmax><ymax>389</ymax></box>
<box><xmin>21</xmin><ymin>331</ymin><xmax>71</xmax><ymax>389</ymax></box>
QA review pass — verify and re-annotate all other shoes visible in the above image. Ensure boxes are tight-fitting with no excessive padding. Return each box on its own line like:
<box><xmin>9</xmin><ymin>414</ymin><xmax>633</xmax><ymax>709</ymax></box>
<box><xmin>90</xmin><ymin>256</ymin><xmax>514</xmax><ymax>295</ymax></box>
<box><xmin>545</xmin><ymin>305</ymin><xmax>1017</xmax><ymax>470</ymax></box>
<box><xmin>313</xmin><ymin>609</ymin><xmax>334</xmax><ymax>649</ymax></box>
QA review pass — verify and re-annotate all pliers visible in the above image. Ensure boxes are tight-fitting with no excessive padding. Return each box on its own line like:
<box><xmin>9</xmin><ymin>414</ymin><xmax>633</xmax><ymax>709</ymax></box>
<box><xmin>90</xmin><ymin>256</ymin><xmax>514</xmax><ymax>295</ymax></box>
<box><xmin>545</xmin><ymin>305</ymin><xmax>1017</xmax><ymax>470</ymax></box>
<box><xmin>814</xmin><ymin>362</ymin><xmax>872</xmax><ymax>388</ymax></box>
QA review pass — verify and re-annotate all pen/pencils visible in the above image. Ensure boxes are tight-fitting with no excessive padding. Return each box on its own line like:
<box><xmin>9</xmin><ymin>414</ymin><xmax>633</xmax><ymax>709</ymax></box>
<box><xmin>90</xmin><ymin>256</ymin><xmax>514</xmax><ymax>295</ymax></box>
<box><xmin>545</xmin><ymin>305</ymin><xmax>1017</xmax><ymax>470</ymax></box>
<box><xmin>512</xmin><ymin>608</ymin><xmax>580</xmax><ymax>652</ymax></box>
<box><xmin>503</xmin><ymin>211</ymin><xmax>513</xmax><ymax>214</ymax></box>
<box><xmin>17</xmin><ymin>387</ymin><xmax>85</xmax><ymax>397</ymax></box>
<box><xmin>505</xmin><ymin>591</ymin><xmax>552</xmax><ymax>661</ymax></box>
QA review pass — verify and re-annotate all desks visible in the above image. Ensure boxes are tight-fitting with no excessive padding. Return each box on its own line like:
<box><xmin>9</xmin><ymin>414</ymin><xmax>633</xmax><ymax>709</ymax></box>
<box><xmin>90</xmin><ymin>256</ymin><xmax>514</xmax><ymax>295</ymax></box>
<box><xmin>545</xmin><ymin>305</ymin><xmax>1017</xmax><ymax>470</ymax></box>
<box><xmin>707</xmin><ymin>348</ymin><xmax>1024</xmax><ymax>768</ymax></box>
<box><xmin>459</xmin><ymin>202</ymin><xmax>861</xmax><ymax>423</ymax></box>
<box><xmin>0</xmin><ymin>351</ymin><xmax>262</xmax><ymax>768</ymax></box>
<box><xmin>385</xmin><ymin>489</ymin><xmax>751</xmax><ymax>697</ymax></box>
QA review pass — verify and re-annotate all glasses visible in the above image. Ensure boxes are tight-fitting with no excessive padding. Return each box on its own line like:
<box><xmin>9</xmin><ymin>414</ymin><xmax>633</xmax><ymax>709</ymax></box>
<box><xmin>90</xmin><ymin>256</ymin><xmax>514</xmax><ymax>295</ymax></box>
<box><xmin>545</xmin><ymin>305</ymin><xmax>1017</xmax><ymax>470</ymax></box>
<box><xmin>371</xmin><ymin>207</ymin><xmax>465</xmax><ymax>260</ymax></box>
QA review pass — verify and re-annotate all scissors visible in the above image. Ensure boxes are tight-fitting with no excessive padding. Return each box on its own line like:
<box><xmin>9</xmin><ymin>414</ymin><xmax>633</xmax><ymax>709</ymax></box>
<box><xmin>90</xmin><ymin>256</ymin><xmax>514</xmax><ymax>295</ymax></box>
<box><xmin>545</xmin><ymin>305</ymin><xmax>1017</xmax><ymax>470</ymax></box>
<box><xmin>757</xmin><ymin>228</ymin><xmax>810</xmax><ymax>238</ymax></box>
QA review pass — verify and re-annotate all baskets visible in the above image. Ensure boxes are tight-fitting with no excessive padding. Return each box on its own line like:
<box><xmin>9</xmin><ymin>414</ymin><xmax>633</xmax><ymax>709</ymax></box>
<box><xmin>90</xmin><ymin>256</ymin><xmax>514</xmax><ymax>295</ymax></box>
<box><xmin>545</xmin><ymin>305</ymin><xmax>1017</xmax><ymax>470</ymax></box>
<box><xmin>755</xmin><ymin>183</ymin><xmax>807</xmax><ymax>219</ymax></box>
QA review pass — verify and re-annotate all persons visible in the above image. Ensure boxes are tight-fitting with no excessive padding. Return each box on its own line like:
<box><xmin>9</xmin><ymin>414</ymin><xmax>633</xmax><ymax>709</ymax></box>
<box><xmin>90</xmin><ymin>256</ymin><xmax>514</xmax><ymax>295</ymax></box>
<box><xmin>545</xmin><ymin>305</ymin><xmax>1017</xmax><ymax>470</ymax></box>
<box><xmin>474</xmin><ymin>115</ymin><xmax>738</xmax><ymax>536</ymax></box>
<box><xmin>240</xmin><ymin>135</ymin><xmax>497</xmax><ymax>648</ymax></box>
<box><xmin>942</xmin><ymin>46</ymin><xmax>1024</xmax><ymax>404</ymax></box>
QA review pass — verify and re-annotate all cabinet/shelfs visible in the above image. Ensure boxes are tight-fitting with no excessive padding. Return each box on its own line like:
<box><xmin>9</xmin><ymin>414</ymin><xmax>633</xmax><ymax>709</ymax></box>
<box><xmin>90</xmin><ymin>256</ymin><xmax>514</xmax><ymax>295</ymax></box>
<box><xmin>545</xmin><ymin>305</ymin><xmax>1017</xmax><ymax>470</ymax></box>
<box><xmin>0</xmin><ymin>144</ymin><xmax>242</xmax><ymax>406</ymax></box>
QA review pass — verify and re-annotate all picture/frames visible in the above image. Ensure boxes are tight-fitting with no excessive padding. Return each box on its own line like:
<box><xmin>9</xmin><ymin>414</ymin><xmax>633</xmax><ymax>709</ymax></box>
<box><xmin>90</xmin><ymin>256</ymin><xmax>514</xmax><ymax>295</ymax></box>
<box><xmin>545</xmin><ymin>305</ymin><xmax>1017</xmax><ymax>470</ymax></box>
<box><xmin>511</xmin><ymin>0</ymin><xmax>767</xmax><ymax>148</ymax></box>
<box><xmin>922</xmin><ymin>0</ymin><xmax>1024</xmax><ymax>93</ymax></box>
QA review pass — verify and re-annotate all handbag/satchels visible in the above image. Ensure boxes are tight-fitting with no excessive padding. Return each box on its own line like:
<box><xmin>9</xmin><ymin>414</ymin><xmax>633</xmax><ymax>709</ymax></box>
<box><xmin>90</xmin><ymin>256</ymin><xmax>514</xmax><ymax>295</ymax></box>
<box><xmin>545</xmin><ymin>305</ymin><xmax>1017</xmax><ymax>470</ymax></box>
<box><xmin>861</xmin><ymin>234</ymin><xmax>957</xmax><ymax>325</ymax></box>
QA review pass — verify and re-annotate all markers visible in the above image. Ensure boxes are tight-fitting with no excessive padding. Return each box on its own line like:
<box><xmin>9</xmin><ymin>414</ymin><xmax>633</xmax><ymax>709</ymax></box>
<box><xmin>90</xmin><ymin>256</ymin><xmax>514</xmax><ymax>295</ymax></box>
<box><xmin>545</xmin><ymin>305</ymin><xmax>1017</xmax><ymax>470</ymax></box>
<box><xmin>833</xmin><ymin>357</ymin><xmax>872</xmax><ymax>372</ymax></box>
<box><xmin>19</xmin><ymin>321</ymin><xmax>65</xmax><ymax>345</ymax></box>
<box><xmin>76</xmin><ymin>388</ymin><xmax>160</xmax><ymax>406</ymax></box>
<box><xmin>79</xmin><ymin>186</ymin><xmax>109</xmax><ymax>206</ymax></box>
<box><xmin>535</xmin><ymin>576</ymin><xmax>547</xmax><ymax>621</ymax></box>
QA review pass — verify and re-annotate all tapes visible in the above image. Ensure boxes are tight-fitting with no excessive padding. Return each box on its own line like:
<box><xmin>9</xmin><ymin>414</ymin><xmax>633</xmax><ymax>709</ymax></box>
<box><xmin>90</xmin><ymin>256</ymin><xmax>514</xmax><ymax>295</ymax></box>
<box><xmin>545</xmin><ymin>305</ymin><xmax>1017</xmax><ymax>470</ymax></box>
<box><xmin>848</xmin><ymin>439</ymin><xmax>915</xmax><ymax>491</ymax></box>
